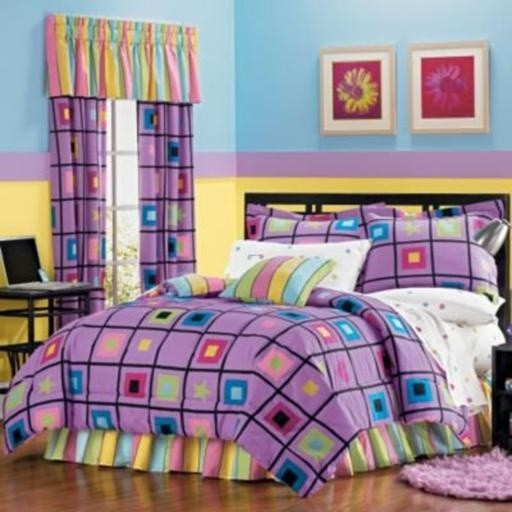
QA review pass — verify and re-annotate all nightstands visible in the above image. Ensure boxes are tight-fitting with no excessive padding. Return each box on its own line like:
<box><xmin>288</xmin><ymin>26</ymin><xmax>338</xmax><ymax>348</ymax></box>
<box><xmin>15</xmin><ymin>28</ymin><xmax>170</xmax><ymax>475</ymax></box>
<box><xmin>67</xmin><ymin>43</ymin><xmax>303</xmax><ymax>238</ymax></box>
<box><xmin>489</xmin><ymin>339</ymin><xmax>512</xmax><ymax>452</ymax></box>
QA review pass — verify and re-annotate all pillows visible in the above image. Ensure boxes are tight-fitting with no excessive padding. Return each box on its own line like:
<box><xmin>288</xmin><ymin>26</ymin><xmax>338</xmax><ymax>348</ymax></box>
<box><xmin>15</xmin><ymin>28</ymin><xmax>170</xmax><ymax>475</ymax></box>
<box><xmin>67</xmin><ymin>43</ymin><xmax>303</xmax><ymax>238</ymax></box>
<box><xmin>355</xmin><ymin>198</ymin><xmax>505</xmax><ymax>304</ymax></box>
<box><xmin>218</xmin><ymin>256</ymin><xmax>339</xmax><ymax>308</ymax></box>
<box><xmin>246</xmin><ymin>201</ymin><xmax>386</xmax><ymax>291</ymax></box>
<box><xmin>223</xmin><ymin>239</ymin><xmax>372</xmax><ymax>291</ymax></box>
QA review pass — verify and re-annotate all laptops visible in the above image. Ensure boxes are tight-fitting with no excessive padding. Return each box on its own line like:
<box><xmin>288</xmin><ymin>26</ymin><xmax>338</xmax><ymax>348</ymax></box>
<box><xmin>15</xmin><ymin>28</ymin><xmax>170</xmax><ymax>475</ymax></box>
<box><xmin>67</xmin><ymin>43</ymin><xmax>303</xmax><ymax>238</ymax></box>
<box><xmin>0</xmin><ymin>234</ymin><xmax>90</xmax><ymax>292</ymax></box>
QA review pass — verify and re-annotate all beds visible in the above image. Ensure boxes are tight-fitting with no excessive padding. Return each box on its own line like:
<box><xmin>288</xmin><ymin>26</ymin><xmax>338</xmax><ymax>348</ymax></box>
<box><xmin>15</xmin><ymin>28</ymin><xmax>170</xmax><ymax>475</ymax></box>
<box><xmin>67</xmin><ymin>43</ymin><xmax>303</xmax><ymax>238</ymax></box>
<box><xmin>2</xmin><ymin>192</ymin><xmax>511</xmax><ymax>499</ymax></box>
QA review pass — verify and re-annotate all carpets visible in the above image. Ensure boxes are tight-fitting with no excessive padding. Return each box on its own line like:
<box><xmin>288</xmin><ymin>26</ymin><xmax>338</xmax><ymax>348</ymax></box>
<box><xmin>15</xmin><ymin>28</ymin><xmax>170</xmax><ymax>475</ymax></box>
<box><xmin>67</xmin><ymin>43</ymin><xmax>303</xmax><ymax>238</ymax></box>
<box><xmin>398</xmin><ymin>445</ymin><xmax>512</xmax><ymax>501</ymax></box>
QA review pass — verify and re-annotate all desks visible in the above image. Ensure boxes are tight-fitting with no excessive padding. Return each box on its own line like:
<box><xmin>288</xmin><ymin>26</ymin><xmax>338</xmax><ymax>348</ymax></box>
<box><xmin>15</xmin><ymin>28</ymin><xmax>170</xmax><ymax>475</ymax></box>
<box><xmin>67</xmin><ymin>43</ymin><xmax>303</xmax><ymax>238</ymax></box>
<box><xmin>0</xmin><ymin>283</ymin><xmax>106</xmax><ymax>378</ymax></box>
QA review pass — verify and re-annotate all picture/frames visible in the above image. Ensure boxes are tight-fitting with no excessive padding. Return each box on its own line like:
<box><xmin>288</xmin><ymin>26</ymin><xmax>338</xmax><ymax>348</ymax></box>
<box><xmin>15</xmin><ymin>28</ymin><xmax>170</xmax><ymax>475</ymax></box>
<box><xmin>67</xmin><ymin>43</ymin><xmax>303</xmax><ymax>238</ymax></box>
<box><xmin>320</xmin><ymin>45</ymin><xmax>397</xmax><ymax>136</ymax></box>
<box><xmin>409</xmin><ymin>41</ymin><xmax>490</xmax><ymax>135</ymax></box>
